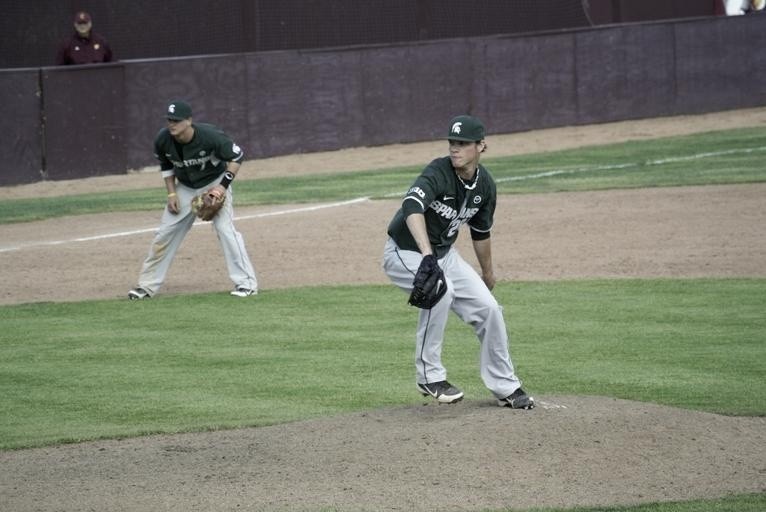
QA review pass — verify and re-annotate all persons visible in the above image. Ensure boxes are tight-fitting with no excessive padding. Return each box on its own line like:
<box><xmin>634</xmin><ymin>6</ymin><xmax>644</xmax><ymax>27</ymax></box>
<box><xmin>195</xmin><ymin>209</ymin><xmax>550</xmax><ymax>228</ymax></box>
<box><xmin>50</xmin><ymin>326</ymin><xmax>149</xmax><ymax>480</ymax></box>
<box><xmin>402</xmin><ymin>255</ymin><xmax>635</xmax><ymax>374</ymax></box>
<box><xmin>381</xmin><ymin>113</ymin><xmax>536</xmax><ymax>411</ymax></box>
<box><xmin>54</xmin><ymin>9</ymin><xmax>113</xmax><ymax>67</ymax></box>
<box><xmin>125</xmin><ymin>98</ymin><xmax>261</xmax><ymax>299</ymax></box>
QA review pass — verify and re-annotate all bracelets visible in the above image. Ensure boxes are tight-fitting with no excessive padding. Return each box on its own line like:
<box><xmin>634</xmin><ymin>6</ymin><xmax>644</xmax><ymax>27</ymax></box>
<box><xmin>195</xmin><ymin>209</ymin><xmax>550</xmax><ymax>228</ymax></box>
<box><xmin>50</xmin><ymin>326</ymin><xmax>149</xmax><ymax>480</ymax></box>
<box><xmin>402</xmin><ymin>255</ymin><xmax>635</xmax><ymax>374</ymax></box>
<box><xmin>220</xmin><ymin>170</ymin><xmax>235</xmax><ymax>189</ymax></box>
<box><xmin>168</xmin><ymin>191</ymin><xmax>177</xmax><ymax>198</ymax></box>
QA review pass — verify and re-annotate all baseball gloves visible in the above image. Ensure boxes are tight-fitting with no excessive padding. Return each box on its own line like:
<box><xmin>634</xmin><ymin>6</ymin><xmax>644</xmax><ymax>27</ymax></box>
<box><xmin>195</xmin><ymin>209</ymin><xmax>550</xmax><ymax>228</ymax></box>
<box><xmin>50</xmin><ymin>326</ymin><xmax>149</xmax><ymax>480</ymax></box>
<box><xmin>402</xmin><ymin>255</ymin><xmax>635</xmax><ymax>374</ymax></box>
<box><xmin>191</xmin><ymin>187</ymin><xmax>224</xmax><ymax>221</ymax></box>
<box><xmin>408</xmin><ymin>255</ymin><xmax>446</xmax><ymax>309</ymax></box>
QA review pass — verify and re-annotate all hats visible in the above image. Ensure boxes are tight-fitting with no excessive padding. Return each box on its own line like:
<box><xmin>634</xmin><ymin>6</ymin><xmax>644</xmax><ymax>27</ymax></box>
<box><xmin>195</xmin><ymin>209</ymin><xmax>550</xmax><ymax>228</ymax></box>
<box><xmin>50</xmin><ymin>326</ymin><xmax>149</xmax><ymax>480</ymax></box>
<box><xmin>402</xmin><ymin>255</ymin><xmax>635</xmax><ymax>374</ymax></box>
<box><xmin>161</xmin><ymin>99</ymin><xmax>191</xmax><ymax>122</ymax></box>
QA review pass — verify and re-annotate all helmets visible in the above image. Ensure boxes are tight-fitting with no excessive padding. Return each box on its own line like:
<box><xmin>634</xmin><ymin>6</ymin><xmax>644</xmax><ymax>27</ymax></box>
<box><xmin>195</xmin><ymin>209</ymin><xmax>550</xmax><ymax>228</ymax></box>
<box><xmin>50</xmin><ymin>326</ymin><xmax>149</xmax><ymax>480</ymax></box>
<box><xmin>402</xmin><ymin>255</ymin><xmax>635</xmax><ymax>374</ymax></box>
<box><xmin>437</xmin><ymin>115</ymin><xmax>486</xmax><ymax>144</ymax></box>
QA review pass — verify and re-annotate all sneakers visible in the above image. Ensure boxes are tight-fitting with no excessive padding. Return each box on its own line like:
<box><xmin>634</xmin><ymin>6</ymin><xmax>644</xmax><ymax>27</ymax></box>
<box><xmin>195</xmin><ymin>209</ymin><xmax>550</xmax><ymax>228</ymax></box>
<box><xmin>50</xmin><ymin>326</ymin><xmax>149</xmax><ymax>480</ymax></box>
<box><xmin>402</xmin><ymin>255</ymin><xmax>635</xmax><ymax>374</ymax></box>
<box><xmin>229</xmin><ymin>288</ymin><xmax>258</xmax><ymax>297</ymax></box>
<box><xmin>414</xmin><ymin>377</ymin><xmax>464</xmax><ymax>404</ymax></box>
<box><xmin>127</xmin><ymin>288</ymin><xmax>153</xmax><ymax>300</ymax></box>
<box><xmin>497</xmin><ymin>388</ymin><xmax>535</xmax><ymax>411</ymax></box>
